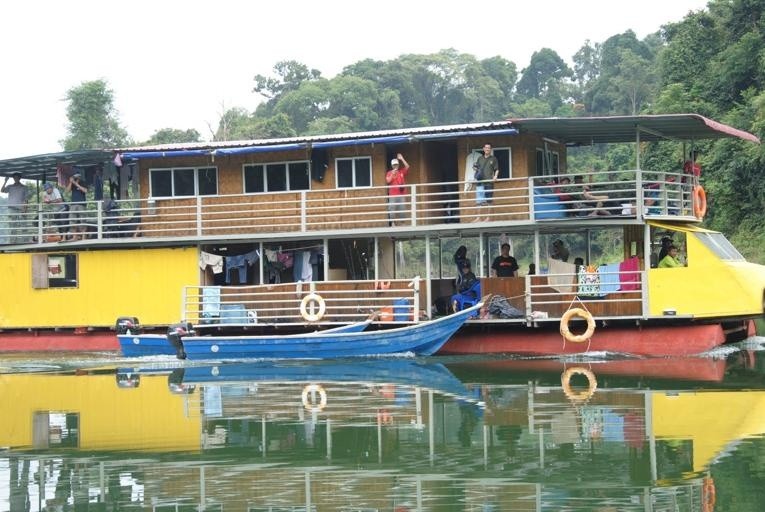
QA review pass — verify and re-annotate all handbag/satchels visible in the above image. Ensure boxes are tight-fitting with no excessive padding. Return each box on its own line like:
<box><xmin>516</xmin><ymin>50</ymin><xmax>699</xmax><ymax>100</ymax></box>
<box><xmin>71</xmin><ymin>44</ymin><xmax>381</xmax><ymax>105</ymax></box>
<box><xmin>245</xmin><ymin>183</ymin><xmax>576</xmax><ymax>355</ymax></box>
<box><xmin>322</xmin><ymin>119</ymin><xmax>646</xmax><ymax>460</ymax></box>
<box><xmin>473</xmin><ymin>169</ymin><xmax>484</xmax><ymax>180</ymax></box>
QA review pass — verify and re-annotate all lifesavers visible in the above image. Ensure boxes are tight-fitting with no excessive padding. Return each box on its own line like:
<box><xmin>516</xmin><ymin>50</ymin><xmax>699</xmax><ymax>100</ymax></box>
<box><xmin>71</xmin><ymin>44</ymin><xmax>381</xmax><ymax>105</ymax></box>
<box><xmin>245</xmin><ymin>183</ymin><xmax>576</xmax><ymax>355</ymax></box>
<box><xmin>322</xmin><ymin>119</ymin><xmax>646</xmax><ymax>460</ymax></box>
<box><xmin>561</xmin><ymin>308</ymin><xmax>596</xmax><ymax>342</ymax></box>
<box><xmin>703</xmin><ymin>475</ymin><xmax>719</xmax><ymax>511</ymax></box>
<box><xmin>299</xmin><ymin>294</ymin><xmax>326</xmax><ymax>321</ymax></box>
<box><xmin>560</xmin><ymin>368</ymin><xmax>598</xmax><ymax>401</ymax></box>
<box><xmin>301</xmin><ymin>384</ymin><xmax>326</xmax><ymax>412</ymax></box>
<box><xmin>691</xmin><ymin>184</ymin><xmax>708</xmax><ymax>217</ymax></box>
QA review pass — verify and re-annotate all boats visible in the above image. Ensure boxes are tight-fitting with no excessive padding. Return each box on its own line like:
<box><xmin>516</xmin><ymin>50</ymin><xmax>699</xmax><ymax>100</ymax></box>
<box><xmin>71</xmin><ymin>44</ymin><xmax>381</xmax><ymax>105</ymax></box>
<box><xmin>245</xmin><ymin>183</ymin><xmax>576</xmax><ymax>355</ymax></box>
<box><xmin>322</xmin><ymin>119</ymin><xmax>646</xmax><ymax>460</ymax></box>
<box><xmin>1</xmin><ymin>105</ymin><xmax>765</xmax><ymax>360</ymax></box>
<box><xmin>114</xmin><ymin>300</ymin><xmax>502</xmax><ymax>364</ymax></box>
<box><xmin>0</xmin><ymin>358</ymin><xmax>765</xmax><ymax>512</ymax></box>
<box><xmin>115</xmin><ymin>361</ymin><xmax>488</xmax><ymax>411</ymax></box>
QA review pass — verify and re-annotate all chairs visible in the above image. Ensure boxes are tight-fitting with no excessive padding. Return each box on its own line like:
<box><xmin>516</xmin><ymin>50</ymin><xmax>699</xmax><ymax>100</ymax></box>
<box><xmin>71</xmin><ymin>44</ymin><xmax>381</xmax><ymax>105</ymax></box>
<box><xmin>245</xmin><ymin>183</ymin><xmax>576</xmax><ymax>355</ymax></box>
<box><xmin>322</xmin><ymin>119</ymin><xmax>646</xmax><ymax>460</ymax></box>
<box><xmin>533</xmin><ymin>185</ymin><xmax>569</xmax><ymax>219</ymax></box>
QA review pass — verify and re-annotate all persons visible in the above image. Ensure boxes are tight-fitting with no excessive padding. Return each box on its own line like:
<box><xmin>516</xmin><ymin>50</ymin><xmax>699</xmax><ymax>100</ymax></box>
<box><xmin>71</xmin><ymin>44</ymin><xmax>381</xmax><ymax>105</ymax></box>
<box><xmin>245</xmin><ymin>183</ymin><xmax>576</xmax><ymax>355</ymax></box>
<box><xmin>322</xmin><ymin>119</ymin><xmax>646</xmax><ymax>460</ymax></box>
<box><xmin>680</xmin><ymin>150</ymin><xmax>701</xmax><ymax>207</ymax></box>
<box><xmin>657</xmin><ymin>244</ymin><xmax>689</xmax><ymax>268</ymax></box>
<box><xmin>658</xmin><ymin>236</ymin><xmax>675</xmax><ymax>261</ymax></box>
<box><xmin>64</xmin><ymin>170</ymin><xmax>89</xmax><ymax>241</ymax></box>
<box><xmin>385</xmin><ymin>152</ymin><xmax>411</xmax><ymax>227</ymax></box>
<box><xmin>1</xmin><ymin>172</ymin><xmax>29</xmax><ymax>244</ymax></box>
<box><xmin>43</xmin><ymin>183</ymin><xmax>71</xmax><ymax>242</ymax></box>
<box><xmin>538</xmin><ymin>176</ymin><xmax>660</xmax><ymax>219</ymax></box>
<box><xmin>573</xmin><ymin>257</ymin><xmax>584</xmax><ymax>272</ymax></box>
<box><xmin>527</xmin><ymin>264</ymin><xmax>536</xmax><ymax>274</ymax></box>
<box><xmin>472</xmin><ymin>141</ymin><xmax>499</xmax><ymax>222</ymax></box>
<box><xmin>453</xmin><ymin>259</ymin><xmax>476</xmax><ymax>297</ymax></box>
<box><xmin>549</xmin><ymin>239</ymin><xmax>570</xmax><ymax>263</ymax></box>
<box><xmin>490</xmin><ymin>243</ymin><xmax>519</xmax><ymax>278</ymax></box>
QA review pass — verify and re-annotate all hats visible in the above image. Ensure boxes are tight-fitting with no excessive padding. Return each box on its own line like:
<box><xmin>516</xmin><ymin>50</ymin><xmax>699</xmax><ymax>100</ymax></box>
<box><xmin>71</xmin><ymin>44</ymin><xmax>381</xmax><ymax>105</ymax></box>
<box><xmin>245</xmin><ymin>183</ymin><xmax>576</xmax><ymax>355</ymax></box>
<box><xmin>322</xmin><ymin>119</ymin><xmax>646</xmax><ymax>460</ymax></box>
<box><xmin>391</xmin><ymin>159</ymin><xmax>399</xmax><ymax>166</ymax></box>
<box><xmin>661</xmin><ymin>236</ymin><xmax>674</xmax><ymax>243</ymax></box>
<box><xmin>43</xmin><ymin>183</ymin><xmax>53</xmax><ymax>191</ymax></box>
